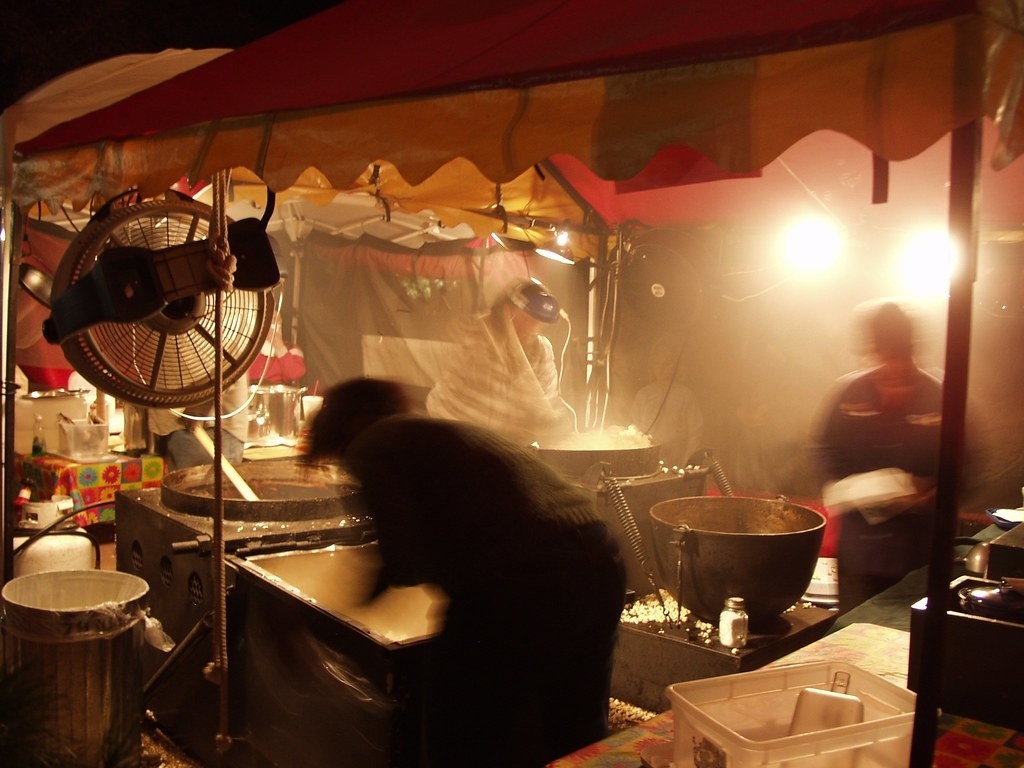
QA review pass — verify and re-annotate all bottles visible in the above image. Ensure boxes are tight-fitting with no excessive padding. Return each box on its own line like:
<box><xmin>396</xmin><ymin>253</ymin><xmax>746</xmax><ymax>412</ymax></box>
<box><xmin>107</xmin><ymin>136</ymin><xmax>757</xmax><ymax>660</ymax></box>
<box><xmin>32</xmin><ymin>414</ymin><xmax>47</xmax><ymax>456</ymax></box>
<box><xmin>720</xmin><ymin>597</ymin><xmax>748</xmax><ymax>646</ymax></box>
<box><xmin>783</xmin><ymin>670</ymin><xmax>865</xmax><ymax>768</ymax></box>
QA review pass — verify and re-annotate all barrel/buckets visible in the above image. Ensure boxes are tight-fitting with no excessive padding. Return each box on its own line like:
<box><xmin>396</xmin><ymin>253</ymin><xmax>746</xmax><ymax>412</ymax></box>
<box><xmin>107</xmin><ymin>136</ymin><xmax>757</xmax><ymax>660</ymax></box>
<box><xmin>248</xmin><ymin>385</ymin><xmax>308</xmax><ymax>441</ymax></box>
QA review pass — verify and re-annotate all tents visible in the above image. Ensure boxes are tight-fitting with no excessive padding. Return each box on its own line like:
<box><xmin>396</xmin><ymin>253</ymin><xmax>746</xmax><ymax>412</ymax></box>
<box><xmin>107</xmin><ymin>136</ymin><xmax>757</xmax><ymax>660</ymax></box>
<box><xmin>6</xmin><ymin>0</ymin><xmax>1024</xmax><ymax>768</ymax></box>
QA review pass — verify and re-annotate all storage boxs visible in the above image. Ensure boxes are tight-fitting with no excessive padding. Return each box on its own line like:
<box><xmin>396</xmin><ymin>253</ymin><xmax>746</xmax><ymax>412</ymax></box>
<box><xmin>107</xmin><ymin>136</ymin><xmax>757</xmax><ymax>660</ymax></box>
<box><xmin>663</xmin><ymin>659</ymin><xmax>943</xmax><ymax>768</ymax></box>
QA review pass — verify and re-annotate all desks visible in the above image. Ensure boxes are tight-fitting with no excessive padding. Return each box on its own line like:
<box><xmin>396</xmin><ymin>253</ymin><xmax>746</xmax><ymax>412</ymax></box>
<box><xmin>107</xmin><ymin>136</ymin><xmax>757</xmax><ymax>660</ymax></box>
<box><xmin>15</xmin><ymin>446</ymin><xmax>166</xmax><ymax>527</ymax></box>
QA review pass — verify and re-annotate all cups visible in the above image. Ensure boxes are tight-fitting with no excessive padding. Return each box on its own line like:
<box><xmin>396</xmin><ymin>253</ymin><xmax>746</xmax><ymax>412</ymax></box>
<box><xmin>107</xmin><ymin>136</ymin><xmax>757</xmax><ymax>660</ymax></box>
<box><xmin>302</xmin><ymin>396</ymin><xmax>323</xmax><ymax>420</ymax></box>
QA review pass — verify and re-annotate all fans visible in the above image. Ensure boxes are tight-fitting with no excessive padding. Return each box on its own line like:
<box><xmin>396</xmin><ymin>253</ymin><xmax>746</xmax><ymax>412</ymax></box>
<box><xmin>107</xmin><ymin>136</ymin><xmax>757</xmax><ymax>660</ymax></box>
<box><xmin>43</xmin><ymin>183</ymin><xmax>287</xmax><ymax>410</ymax></box>
<box><xmin>622</xmin><ymin>239</ymin><xmax>703</xmax><ymax>327</ymax></box>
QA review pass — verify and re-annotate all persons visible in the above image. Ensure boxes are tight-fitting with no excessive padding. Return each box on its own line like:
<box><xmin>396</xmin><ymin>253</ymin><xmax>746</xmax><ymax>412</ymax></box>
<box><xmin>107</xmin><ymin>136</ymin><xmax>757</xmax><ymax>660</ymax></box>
<box><xmin>813</xmin><ymin>302</ymin><xmax>987</xmax><ymax>615</ymax></box>
<box><xmin>123</xmin><ymin>375</ymin><xmax>250</xmax><ymax>474</ymax></box>
<box><xmin>300</xmin><ymin>379</ymin><xmax>625</xmax><ymax>768</ymax></box>
<box><xmin>424</xmin><ymin>277</ymin><xmax>567</xmax><ymax>449</ymax></box>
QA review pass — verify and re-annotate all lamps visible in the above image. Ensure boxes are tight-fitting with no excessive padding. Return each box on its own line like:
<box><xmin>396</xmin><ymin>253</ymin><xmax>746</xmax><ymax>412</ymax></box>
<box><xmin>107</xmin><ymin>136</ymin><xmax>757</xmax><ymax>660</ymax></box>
<box><xmin>535</xmin><ymin>217</ymin><xmax>576</xmax><ymax>265</ymax></box>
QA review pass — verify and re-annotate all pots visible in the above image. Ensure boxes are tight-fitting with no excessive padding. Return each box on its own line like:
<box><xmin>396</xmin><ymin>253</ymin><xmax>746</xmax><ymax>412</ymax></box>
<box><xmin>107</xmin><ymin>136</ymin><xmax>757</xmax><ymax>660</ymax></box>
<box><xmin>536</xmin><ymin>439</ymin><xmax>663</xmax><ymax>476</ymax></box>
